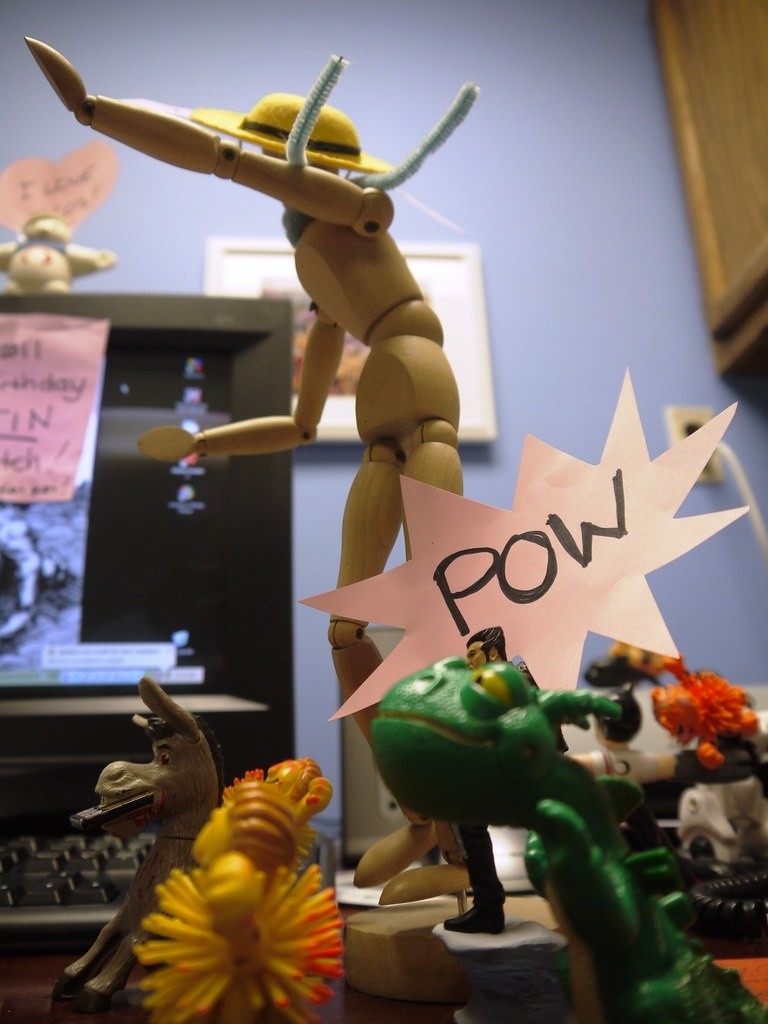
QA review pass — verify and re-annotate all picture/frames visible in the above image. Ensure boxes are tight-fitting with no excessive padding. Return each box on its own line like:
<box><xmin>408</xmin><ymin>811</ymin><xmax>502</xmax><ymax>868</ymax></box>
<box><xmin>206</xmin><ymin>235</ymin><xmax>496</xmax><ymax>442</ymax></box>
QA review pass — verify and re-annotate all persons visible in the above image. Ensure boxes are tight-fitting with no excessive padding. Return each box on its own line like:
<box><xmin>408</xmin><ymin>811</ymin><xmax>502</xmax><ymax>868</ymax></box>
<box><xmin>571</xmin><ymin>690</ymin><xmax>675</xmax><ymax>780</ymax></box>
<box><xmin>22</xmin><ymin>36</ymin><xmax>464</xmax><ymax>905</ymax></box>
<box><xmin>443</xmin><ymin>626</ymin><xmax>507</xmax><ymax>933</ymax></box>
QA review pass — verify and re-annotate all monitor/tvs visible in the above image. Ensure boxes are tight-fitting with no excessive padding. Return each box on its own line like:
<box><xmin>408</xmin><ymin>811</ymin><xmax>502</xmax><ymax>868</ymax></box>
<box><xmin>0</xmin><ymin>290</ymin><xmax>295</xmax><ymax>838</ymax></box>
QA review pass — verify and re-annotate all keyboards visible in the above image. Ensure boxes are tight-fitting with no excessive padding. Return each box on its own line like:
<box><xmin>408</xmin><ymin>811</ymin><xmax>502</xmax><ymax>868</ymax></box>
<box><xmin>0</xmin><ymin>832</ymin><xmax>336</xmax><ymax>955</ymax></box>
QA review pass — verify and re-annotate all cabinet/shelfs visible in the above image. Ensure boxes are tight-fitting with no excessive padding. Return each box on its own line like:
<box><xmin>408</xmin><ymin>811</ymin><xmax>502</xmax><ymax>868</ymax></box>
<box><xmin>654</xmin><ymin>0</ymin><xmax>768</xmax><ymax>375</ymax></box>
